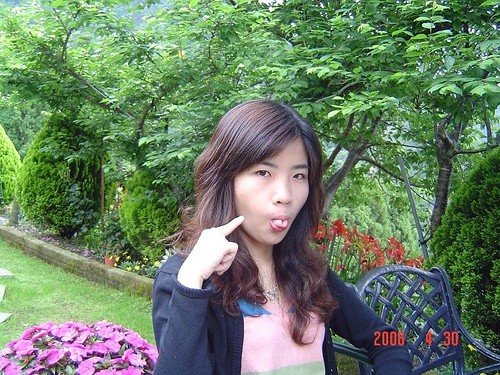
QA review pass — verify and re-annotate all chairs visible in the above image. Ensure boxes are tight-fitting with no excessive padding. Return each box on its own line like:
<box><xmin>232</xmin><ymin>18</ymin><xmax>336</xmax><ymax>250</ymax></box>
<box><xmin>343</xmin><ymin>265</ymin><xmax>500</xmax><ymax>375</ymax></box>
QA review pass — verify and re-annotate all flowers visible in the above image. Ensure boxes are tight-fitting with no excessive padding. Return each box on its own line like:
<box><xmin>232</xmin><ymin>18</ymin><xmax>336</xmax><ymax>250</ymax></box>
<box><xmin>0</xmin><ymin>319</ymin><xmax>159</xmax><ymax>375</ymax></box>
<box><xmin>312</xmin><ymin>219</ymin><xmax>424</xmax><ymax>281</ymax></box>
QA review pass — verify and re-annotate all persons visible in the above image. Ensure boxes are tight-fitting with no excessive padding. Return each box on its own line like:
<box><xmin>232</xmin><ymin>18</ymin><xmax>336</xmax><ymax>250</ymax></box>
<box><xmin>152</xmin><ymin>98</ymin><xmax>412</xmax><ymax>375</ymax></box>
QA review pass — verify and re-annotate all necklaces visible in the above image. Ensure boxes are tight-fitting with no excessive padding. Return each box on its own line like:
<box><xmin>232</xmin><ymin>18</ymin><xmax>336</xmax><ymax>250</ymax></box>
<box><xmin>260</xmin><ymin>282</ymin><xmax>280</xmax><ymax>301</ymax></box>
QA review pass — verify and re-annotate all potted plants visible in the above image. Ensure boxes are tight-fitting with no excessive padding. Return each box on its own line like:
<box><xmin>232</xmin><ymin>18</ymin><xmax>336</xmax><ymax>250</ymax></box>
<box><xmin>105</xmin><ymin>245</ymin><xmax>117</xmax><ymax>267</ymax></box>
